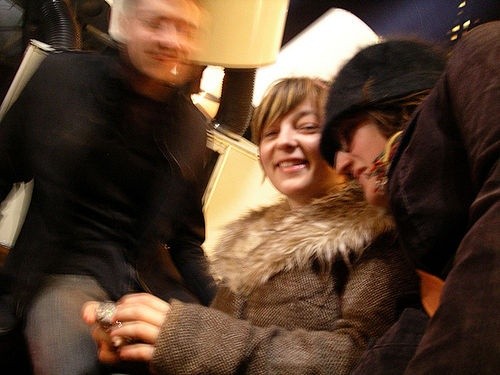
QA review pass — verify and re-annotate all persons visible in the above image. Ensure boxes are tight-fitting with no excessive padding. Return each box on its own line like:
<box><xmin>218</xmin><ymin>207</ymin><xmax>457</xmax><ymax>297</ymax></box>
<box><xmin>320</xmin><ymin>19</ymin><xmax>500</xmax><ymax>375</ymax></box>
<box><xmin>83</xmin><ymin>78</ymin><xmax>408</xmax><ymax>375</ymax></box>
<box><xmin>1</xmin><ymin>1</ymin><xmax>220</xmax><ymax>375</ymax></box>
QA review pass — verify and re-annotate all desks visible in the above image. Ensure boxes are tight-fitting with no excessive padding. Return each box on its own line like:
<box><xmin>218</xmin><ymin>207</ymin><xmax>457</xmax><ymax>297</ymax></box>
<box><xmin>197</xmin><ymin>127</ymin><xmax>288</xmax><ymax>257</ymax></box>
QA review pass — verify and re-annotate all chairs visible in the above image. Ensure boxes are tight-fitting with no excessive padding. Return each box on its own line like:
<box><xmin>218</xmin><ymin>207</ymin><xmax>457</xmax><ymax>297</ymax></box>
<box><xmin>0</xmin><ymin>38</ymin><xmax>56</xmax><ymax>249</ymax></box>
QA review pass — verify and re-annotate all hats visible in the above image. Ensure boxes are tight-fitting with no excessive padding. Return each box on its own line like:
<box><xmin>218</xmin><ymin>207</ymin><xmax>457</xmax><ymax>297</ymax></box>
<box><xmin>317</xmin><ymin>38</ymin><xmax>450</xmax><ymax>169</ymax></box>
